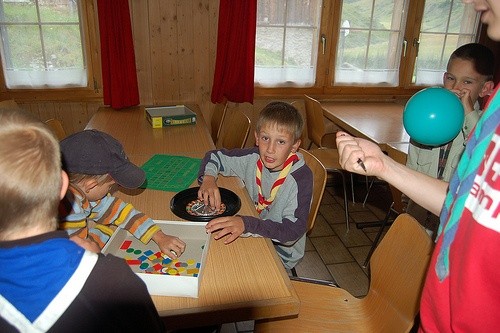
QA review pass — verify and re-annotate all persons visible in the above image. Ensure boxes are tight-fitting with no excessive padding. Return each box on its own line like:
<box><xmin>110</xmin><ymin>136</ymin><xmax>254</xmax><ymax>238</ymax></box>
<box><xmin>58</xmin><ymin>129</ymin><xmax>187</xmax><ymax>260</ymax></box>
<box><xmin>399</xmin><ymin>43</ymin><xmax>500</xmax><ymax>245</ymax></box>
<box><xmin>0</xmin><ymin>117</ymin><xmax>169</xmax><ymax>333</ymax></box>
<box><xmin>335</xmin><ymin>0</ymin><xmax>500</xmax><ymax>333</ymax></box>
<box><xmin>197</xmin><ymin>102</ymin><xmax>315</xmax><ymax>271</ymax></box>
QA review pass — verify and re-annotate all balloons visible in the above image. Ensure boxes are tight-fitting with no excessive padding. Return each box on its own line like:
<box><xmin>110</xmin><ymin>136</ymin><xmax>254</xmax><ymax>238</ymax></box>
<box><xmin>403</xmin><ymin>87</ymin><xmax>465</xmax><ymax>147</ymax></box>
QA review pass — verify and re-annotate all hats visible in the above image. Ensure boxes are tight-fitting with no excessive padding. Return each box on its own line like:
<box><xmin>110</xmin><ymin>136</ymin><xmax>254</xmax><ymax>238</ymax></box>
<box><xmin>59</xmin><ymin>129</ymin><xmax>145</xmax><ymax>189</ymax></box>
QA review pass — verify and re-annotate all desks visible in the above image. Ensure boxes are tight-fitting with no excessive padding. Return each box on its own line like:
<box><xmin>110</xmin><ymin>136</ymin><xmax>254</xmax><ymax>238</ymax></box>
<box><xmin>318</xmin><ymin>101</ymin><xmax>408</xmax><ymax>207</ymax></box>
<box><xmin>83</xmin><ymin>102</ymin><xmax>299</xmax><ymax>330</ymax></box>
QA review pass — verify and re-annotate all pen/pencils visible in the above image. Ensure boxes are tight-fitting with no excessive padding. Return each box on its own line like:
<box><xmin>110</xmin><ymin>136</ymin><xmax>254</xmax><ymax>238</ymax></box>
<box><xmin>340</xmin><ymin>133</ymin><xmax>368</xmax><ymax>173</ymax></box>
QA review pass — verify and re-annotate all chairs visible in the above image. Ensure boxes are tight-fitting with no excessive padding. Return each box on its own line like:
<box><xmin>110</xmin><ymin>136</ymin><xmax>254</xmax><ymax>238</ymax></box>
<box><xmin>253</xmin><ymin>213</ymin><xmax>435</xmax><ymax>333</ymax></box>
<box><xmin>290</xmin><ymin>95</ymin><xmax>369</xmax><ymax>233</ymax></box>
<box><xmin>0</xmin><ymin>98</ymin><xmax>67</xmax><ymax>143</ymax></box>
<box><xmin>215</xmin><ymin>101</ymin><xmax>251</xmax><ymax>152</ymax></box>
<box><xmin>291</xmin><ymin>148</ymin><xmax>327</xmax><ymax>278</ymax></box>
<box><xmin>363</xmin><ymin>148</ymin><xmax>428</xmax><ymax>269</ymax></box>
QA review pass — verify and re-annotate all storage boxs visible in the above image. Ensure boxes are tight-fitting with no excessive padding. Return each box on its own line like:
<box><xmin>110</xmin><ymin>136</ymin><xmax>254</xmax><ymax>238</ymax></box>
<box><xmin>144</xmin><ymin>104</ymin><xmax>196</xmax><ymax>128</ymax></box>
<box><xmin>100</xmin><ymin>220</ymin><xmax>212</xmax><ymax>299</ymax></box>
